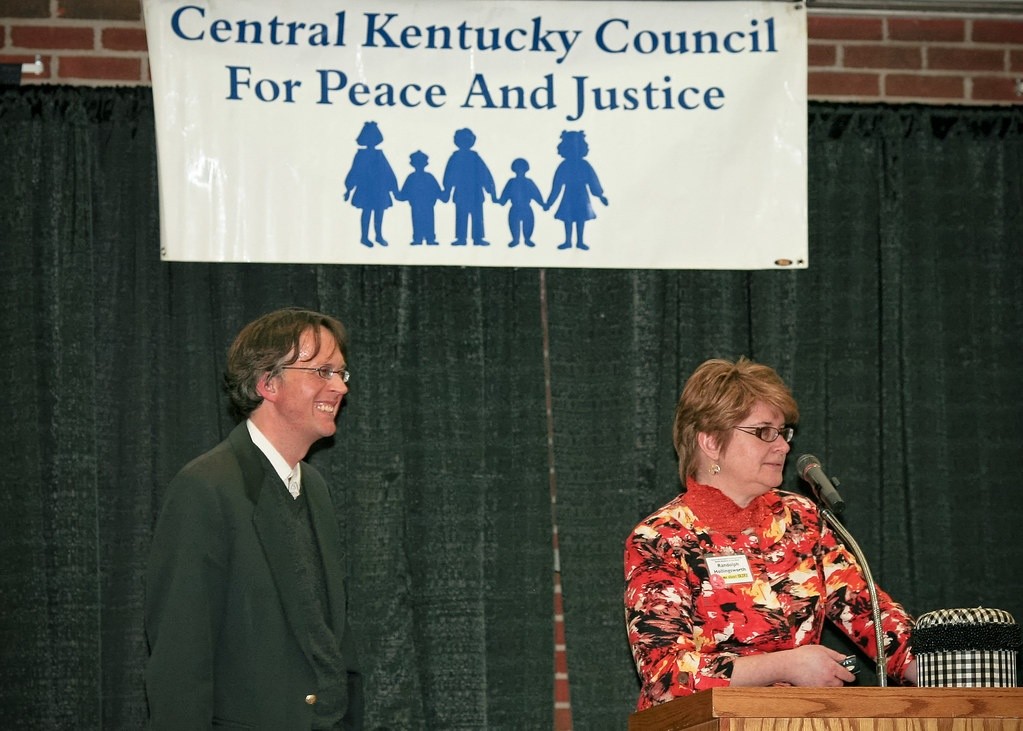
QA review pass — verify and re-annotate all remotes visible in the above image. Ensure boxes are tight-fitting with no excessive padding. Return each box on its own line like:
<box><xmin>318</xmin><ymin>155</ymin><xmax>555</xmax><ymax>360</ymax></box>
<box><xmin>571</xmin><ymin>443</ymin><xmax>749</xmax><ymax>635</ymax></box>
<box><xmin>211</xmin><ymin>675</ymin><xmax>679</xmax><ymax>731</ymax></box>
<box><xmin>836</xmin><ymin>655</ymin><xmax>860</xmax><ymax>675</ymax></box>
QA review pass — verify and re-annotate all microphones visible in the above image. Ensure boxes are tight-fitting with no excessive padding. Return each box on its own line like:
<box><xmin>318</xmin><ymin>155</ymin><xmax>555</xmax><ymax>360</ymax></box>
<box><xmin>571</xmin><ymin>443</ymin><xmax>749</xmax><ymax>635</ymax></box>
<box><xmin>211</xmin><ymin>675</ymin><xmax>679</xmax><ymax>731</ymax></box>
<box><xmin>797</xmin><ymin>454</ymin><xmax>845</xmax><ymax>515</ymax></box>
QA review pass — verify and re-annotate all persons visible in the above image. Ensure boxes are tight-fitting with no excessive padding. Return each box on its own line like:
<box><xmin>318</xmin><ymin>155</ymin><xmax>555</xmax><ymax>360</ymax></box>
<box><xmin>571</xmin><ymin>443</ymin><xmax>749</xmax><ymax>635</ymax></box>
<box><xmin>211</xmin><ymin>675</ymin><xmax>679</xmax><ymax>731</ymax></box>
<box><xmin>623</xmin><ymin>357</ymin><xmax>919</xmax><ymax>712</ymax></box>
<box><xmin>143</xmin><ymin>307</ymin><xmax>366</xmax><ymax>731</ymax></box>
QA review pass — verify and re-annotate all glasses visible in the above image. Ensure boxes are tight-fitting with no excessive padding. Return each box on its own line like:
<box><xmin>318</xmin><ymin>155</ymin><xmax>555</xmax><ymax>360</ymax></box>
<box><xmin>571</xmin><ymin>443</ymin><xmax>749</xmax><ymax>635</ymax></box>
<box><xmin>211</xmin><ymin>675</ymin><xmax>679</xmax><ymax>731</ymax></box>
<box><xmin>271</xmin><ymin>364</ymin><xmax>349</xmax><ymax>383</ymax></box>
<box><xmin>731</xmin><ymin>425</ymin><xmax>794</xmax><ymax>443</ymax></box>
